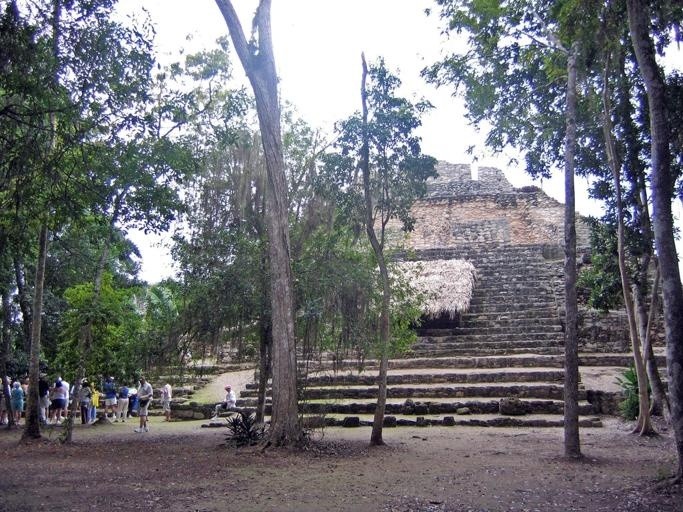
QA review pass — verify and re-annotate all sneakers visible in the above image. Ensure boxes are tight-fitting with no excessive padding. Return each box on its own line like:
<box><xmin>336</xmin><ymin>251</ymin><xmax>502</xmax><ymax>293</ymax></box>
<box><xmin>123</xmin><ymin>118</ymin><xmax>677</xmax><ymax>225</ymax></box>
<box><xmin>46</xmin><ymin>420</ymin><xmax>67</xmax><ymax>425</ymax></box>
<box><xmin>112</xmin><ymin>416</ymin><xmax>126</xmax><ymax>422</ymax></box>
<box><xmin>134</xmin><ymin>428</ymin><xmax>149</xmax><ymax>433</ymax></box>
<box><xmin>0</xmin><ymin>421</ymin><xmax>6</xmax><ymax>425</ymax></box>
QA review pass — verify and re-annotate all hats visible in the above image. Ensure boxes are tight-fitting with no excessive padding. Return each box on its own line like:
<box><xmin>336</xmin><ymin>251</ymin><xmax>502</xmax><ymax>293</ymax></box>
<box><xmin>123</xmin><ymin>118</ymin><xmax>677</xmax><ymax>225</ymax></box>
<box><xmin>40</xmin><ymin>372</ymin><xmax>47</xmax><ymax>378</ymax></box>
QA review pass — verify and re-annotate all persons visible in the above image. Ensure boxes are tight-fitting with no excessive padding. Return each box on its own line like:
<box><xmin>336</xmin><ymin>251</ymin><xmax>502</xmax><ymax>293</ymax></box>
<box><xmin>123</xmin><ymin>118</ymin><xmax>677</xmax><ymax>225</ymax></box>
<box><xmin>210</xmin><ymin>385</ymin><xmax>236</xmax><ymax>420</ymax></box>
<box><xmin>0</xmin><ymin>371</ymin><xmax>152</xmax><ymax>432</ymax></box>
<box><xmin>161</xmin><ymin>379</ymin><xmax>173</xmax><ymax>421</ymax></box>
